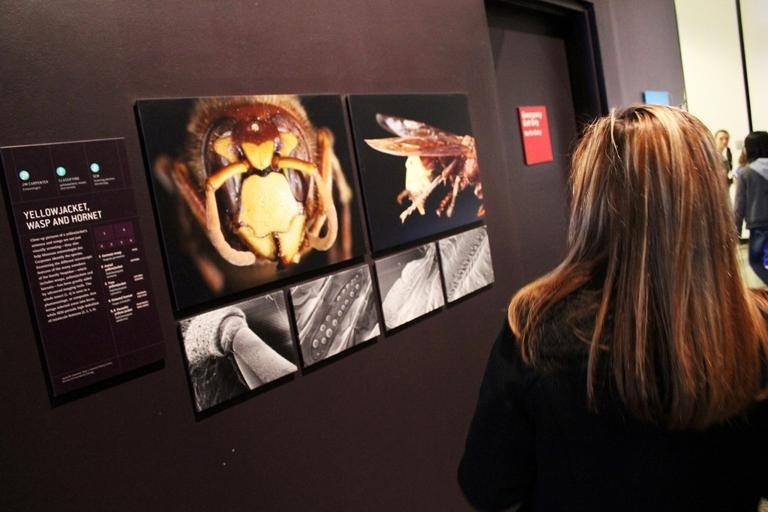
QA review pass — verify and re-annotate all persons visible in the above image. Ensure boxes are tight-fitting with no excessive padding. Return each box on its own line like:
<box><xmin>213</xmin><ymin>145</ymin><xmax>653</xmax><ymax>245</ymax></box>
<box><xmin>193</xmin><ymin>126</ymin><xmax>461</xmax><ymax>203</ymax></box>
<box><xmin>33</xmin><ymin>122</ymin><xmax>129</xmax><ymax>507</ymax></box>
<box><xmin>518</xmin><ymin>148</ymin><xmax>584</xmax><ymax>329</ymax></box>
<box><xmin>454</xmin><ymin>101</ymin><xmax>767</xmax><ymax>512</ymax></box>
<box><xmin>734</xmin><ymin>132</ymin><xmax>767</xmax><ymax>291</ymax></box>
<box><xmin>735</xmin><ymin>147</ymin><xmax>767</xmax><ymax>270</ymax></box>
<box><xmin>713</xmin><ymin>129</ymin><xmax>736</xmax><ymax>190</ymax></box>
